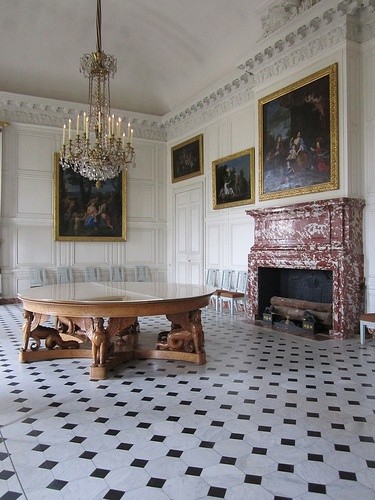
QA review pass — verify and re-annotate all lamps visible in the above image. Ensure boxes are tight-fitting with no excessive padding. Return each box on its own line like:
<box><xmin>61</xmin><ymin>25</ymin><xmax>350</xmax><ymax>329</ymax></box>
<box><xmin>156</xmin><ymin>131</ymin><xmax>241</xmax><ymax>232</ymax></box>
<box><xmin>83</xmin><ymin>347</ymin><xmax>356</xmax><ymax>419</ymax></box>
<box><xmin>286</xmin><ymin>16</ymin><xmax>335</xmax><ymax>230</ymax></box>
<box><xmin>58</xmin><ymin>2</ymin><xmax>138</xmax><ymax>181</ymax></box>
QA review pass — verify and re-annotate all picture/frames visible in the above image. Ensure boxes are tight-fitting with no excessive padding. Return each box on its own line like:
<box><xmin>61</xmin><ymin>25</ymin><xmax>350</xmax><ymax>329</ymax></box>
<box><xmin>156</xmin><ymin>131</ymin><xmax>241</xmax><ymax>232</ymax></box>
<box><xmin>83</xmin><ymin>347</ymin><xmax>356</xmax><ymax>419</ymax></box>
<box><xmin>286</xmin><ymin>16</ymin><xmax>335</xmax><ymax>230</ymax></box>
<box><xmin>54</xmin><ymin>150</ymin><xmax>127</xmax><ymax>242</ymax></box>
<box><xmin>168</xmin><ymin>133</ymin><xmax>206</xmax><ymax>184</ymax></box>
<box><xmin>256</xmin><ymin>61</ymin><xmax>342</xmax><ymax>201</ymax></box>
<box><xmin>209</xmin><ymin>147</ymin><xmax>255</xmax><ymax>210</ymax></box>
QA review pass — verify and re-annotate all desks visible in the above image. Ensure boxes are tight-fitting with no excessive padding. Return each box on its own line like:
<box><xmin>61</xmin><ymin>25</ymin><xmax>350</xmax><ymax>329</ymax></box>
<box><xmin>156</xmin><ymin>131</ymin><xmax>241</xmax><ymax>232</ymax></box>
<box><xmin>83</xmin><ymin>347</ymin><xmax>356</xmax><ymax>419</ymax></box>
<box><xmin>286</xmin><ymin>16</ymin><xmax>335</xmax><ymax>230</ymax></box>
<box><xmin>14</xmin><ymin>277</ymin><xmax>218</xmax><ymax>381</ymax></box>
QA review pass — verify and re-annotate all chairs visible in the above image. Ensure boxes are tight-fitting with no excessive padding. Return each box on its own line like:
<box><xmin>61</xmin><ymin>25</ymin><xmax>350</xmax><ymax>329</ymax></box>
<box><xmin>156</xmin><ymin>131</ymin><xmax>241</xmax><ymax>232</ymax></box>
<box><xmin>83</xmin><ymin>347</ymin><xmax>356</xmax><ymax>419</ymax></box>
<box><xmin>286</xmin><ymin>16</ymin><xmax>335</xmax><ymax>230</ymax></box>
<box><xmin>203</xmin><ymin>266</ymin><xmax>250</xmax><ymax>317</ymax></box>
<box><xmin>28</xmin><ymin>268</ymin><xmax>43</xmax><ymax>287</ymax></box>
<box><xmin>55</xmin><ymin>267</ymin><xmax>74</xmax><ymax>284</ymax></box>
<box><xmin>135</xmin><ymin>266</ymin><xmax>149</xmax><ymax>282</ymax></box>
<box><xmin>85</xmin><ymin>267</ymin><xmax>101</xmax><ymax>282</ymax></box>
<box><xmin>360</xmin><ymin>299</ymin><xmax>375</xmax><ymax>342</ymax></box>
<box><xmin>110</xmin><ymin>266</ymin><xmax>124</xmax><ymax>282</ymax></box>
